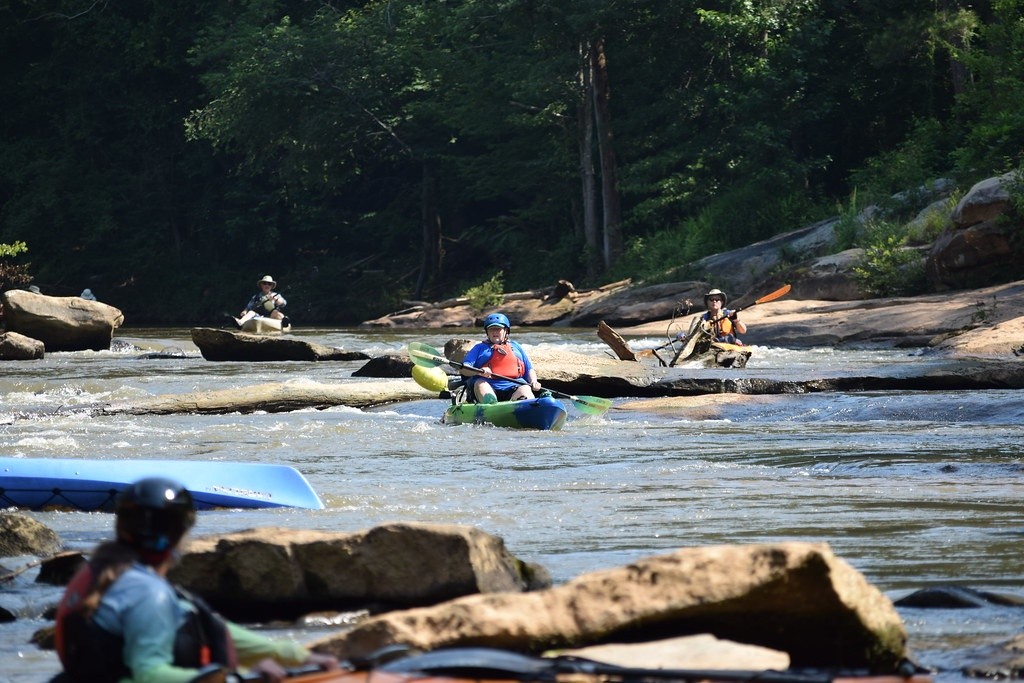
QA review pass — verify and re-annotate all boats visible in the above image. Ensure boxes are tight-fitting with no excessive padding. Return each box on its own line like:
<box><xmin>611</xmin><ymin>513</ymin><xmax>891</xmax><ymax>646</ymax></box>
<box><xmin>444</xmin><ymin>392</ymin><xmax>567</xmax><ymax>431</ymax></box>
<box><xmin>241</xmin><ymin>316</ymin><xmax>292</xmax><ymax>334</ymax></box>
<box><xmin>708</xmin><ymin>342</ymin><xmax>753</xmax><ymax>369</ymax></box>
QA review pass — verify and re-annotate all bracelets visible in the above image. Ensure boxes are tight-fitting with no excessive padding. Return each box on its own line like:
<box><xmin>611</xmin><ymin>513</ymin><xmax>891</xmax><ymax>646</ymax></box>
<box><xmin>735</xmin><ymin>320</ymin><xmax>741</xmax><ymax>325</ymax></box>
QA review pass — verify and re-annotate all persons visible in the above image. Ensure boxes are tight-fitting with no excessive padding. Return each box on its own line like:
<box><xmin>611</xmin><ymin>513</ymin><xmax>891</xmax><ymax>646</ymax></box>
<box><xmin>458</xmin><ymin>314</ymin><xmax>541</xmax><ymax>403</ymax></box>
<box><xmin>229</xmin><ymin>275</ymin><xmax>290</xmax><ymax>330</ymax></box>
<box><xmin>676</xmin><ymin>289</ymin><xmax>747</xmax><ymax>346</ymax></box>
<box><xmin>80</xmin><ymin>289</ymin><xmax>96</xmax><ymax>301</ymax></box>
<box><xmin>53</xmin><ymin>479</ymin><xmax>339</xmax><ymax>683</ymax></box>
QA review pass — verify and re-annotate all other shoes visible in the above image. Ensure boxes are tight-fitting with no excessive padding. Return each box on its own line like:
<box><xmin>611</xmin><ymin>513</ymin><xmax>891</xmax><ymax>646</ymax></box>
<box><xmin>231</xmin><ymin>316</ymin><xmax>243</xmax><ymax>331</ymax></box>
<box><xmin>282</xmin><ymin>316</ymin><xmax>290</xmax><ymax>327</ymax></box>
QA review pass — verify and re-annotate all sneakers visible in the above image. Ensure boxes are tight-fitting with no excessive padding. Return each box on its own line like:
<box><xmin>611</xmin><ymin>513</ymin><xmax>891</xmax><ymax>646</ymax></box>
<box><xmin>483</xmin><ymin>393</ymin><xmax>497</xmax><ymax>404</ymax></box>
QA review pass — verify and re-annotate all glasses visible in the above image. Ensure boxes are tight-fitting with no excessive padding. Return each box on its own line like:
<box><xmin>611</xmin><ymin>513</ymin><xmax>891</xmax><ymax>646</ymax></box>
<box><xmin>708</xmin><ymin>297</ymin><xmax>723</xmax><ymax>302</ymax></box>
<box><xmin>488</xmin><ymin>328</ymin><xmax>505</xmax><ymax>333</ymax></box>
<box><xmin>262</xmin><ymin>282</ymin><xmax>273</xmax><ymax>286</ymax></box>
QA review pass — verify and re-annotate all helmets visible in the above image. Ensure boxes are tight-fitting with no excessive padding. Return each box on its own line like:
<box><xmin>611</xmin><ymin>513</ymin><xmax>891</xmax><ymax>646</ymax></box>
<box><xmin>116</xmin><ymin>478</ymin><xmax>196</xmax><ymax>538</ymax></box>
<box><xmin>484</xmin><ymin>312</ymin><xmax>510</xmax><ymax>336</ymax></box>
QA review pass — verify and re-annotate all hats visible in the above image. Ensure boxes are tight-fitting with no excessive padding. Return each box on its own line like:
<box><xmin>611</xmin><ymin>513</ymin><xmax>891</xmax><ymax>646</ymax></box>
<box><xmin>257</xmin><ymin>275</ymin><xmax>276</xmax><ymax>289</ymax></box>
<box><xmin>25</xmin><ymin>285</ymin><xmax>43</xmax><ymax>296</ymax></box>
<box><xmin>81</xmin><ymin>288</ymin><xmax>93</xmax><ymax>300</ymax></box>
<box><xmin>704</xmin><ymin>289</ymin><xmax>728</xmax><ymax>310</ymax></box>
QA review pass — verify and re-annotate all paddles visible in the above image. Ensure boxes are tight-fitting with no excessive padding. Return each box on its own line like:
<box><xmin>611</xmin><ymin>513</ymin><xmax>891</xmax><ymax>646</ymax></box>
<box><xmin>636</xmin><ymin>284</ymin><xmax>791</xmax><ymax>358</ymax></box>
<box><xmin>406</xmin><ymin>340</ymin><xmax>612</xmax><ymax>416</ymax></box>
